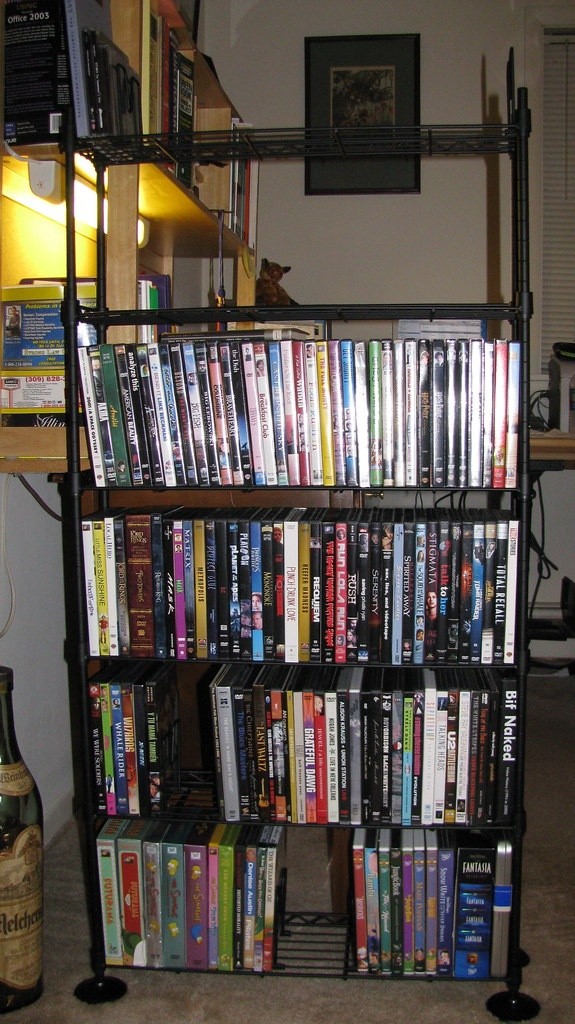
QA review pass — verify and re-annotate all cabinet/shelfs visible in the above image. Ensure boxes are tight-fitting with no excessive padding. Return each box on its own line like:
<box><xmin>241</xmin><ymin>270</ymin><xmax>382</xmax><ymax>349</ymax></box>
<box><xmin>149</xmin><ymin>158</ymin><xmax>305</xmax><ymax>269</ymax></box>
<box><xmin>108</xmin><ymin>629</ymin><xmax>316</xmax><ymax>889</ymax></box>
<box><xmin>61</xmin><ymin>86</ymin><xmax>546</xmax><ymax>1023</ymax></box>
<box><xmin>0</xmin><ymin>0</ymin><xmax>259</xmax><ymax>474</ymax></box>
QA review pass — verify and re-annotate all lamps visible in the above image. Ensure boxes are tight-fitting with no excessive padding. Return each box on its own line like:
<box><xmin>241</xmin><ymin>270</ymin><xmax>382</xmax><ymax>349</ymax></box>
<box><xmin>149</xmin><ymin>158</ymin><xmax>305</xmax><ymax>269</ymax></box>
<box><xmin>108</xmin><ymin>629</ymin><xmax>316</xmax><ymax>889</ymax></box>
<box><xmin>28</xmin><ymin>160</ymin><xmax>150</xmax><ymax>248</ymax></box>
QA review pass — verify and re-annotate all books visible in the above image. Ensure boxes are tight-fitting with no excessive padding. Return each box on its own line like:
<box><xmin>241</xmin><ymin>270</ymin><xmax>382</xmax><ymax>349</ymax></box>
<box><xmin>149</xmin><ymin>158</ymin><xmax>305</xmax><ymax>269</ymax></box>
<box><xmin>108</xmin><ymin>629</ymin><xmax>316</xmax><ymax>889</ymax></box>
<box><xmin>75</xmin><ymin>321</ymin><xmax>523</xmax><ymax>984</ymax></box>
<box><xmin>1</xmin><ymin>0</ymin><xmax>259</xmax><ymax>252</ymax></box>
<box><xmin>0</xmin><ymin>273</ymin><xmax>172</xmax><ymax>461</ymax></box>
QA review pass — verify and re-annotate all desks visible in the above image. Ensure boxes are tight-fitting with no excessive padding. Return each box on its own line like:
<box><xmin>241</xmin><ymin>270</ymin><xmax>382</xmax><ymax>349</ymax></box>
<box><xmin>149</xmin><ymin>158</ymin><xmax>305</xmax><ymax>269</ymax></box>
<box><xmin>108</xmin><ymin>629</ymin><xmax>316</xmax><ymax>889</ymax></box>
<box><xmin>531</xmin><ymin>432</ymin><xmax>575</xmax><ymax>679</ymax></box>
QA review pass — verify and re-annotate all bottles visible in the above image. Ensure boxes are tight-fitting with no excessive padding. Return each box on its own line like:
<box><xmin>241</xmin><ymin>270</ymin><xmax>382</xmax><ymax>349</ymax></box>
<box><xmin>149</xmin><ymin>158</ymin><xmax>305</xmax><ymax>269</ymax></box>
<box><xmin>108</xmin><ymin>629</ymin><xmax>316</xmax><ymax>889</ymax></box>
<box><xmin>0</xmin><ymin>665</ymin><xmax>46</xmax><ymax>1013</ymax></box>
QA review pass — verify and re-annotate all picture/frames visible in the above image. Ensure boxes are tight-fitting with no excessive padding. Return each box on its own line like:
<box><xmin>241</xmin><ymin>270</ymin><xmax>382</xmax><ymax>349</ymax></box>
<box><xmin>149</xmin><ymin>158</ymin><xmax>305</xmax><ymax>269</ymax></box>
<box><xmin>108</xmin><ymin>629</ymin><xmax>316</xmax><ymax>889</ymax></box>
<box><xmin>305</xmin><ymin>32</ymin><xmax>422</xmax><ymax>193</ymax></box>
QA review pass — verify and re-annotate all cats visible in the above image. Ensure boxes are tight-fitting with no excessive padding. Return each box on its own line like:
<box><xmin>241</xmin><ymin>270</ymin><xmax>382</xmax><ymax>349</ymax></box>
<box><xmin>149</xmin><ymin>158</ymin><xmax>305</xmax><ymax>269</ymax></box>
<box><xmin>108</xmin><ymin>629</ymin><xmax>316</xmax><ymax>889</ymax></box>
<box><xmin>256</xmin><ymin>258</ymin><xmax>300</xmax><ymax>306</ymax></box>
